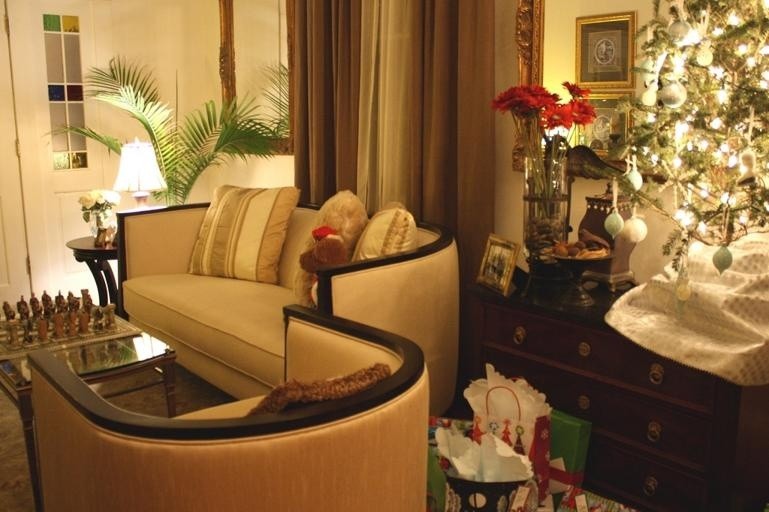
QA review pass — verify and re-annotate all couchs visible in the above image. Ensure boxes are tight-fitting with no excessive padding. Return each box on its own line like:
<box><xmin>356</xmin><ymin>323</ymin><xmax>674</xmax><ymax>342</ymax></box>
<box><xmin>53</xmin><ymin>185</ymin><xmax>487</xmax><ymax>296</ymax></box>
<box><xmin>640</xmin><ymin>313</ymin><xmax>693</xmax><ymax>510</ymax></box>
<box><xmin>114</xmin><ymin>202</ymin><xmax>461</xmax><ymax>416</ymax></box>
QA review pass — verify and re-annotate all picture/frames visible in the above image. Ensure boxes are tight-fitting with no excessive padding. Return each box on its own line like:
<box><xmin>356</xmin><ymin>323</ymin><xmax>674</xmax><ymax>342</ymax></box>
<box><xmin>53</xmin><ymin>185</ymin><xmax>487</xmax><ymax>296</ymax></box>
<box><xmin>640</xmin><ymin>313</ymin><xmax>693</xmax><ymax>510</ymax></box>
<box><xmin>475</xmin><ymin>232</ymin><xmax>521</xmax><ymax>297</ymax></box>
<box><xmin>574</xmin><ymin>10</ymin><xmax>638</xmax><ymax>159</ymax></box>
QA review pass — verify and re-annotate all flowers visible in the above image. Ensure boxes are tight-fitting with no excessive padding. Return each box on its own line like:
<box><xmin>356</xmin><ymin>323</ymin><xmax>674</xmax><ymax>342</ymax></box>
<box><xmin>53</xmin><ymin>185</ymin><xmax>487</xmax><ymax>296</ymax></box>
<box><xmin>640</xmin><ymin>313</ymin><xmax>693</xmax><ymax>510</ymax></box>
<box><xmin>490</xmin><ymin>78</ymin><xmax>597</xmax><ymax>243</ymax></box>
<box><xmin>78</xmin><ymin>191</ymin><xmax>118</xmax><ymax>233</ymax></box>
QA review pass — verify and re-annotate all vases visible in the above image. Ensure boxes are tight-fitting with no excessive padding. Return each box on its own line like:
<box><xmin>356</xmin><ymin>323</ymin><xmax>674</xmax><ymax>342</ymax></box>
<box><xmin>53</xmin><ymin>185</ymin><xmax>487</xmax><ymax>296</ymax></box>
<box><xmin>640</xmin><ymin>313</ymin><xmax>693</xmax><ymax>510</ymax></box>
<box><xmin>94</xmin><ymin>227</ymin><xmax>110</xmax><ymax>249</ymax></box>
<box><xmin>522</xmin><ymin>155</ymin><xmax>571</xmax><ymax>253</ymax></box>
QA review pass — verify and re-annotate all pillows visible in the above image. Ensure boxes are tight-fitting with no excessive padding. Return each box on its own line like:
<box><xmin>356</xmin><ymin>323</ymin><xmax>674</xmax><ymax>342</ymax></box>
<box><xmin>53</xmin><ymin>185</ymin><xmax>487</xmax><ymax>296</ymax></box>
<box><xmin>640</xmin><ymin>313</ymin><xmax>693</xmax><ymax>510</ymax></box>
<box><xmin>187</xmin><ymin>184</ymin><xmax>421</xmax><ymax>309</ymax></box>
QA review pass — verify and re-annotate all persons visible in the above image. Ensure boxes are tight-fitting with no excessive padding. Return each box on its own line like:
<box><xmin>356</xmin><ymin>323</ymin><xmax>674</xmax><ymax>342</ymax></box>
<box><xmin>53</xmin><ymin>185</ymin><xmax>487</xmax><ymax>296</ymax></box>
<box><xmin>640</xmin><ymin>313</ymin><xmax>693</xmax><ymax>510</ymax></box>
<box><xmin>2</xmin><ymin>288</ymin><xmax>118</xmax><ymax>352</ymax></box>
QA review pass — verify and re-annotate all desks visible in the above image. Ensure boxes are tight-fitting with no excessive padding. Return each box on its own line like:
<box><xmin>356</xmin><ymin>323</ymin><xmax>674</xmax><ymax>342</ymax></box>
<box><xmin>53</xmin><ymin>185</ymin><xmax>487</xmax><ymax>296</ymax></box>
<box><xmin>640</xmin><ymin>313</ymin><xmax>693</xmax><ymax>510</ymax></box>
<box><xmin>65</xmin><ymin>237</ymin><xmax>119</xmax><ymax>307</ymax></box>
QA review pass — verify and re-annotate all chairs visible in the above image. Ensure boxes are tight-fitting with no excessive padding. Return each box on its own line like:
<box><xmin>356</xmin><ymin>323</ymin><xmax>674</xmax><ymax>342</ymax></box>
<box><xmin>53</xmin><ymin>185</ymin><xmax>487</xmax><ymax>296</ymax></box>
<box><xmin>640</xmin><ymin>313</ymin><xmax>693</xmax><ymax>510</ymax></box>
<box><xmin>25</xmin><ymin>304</ymin><xmax>432</xmax><ymax>512</ymax></box>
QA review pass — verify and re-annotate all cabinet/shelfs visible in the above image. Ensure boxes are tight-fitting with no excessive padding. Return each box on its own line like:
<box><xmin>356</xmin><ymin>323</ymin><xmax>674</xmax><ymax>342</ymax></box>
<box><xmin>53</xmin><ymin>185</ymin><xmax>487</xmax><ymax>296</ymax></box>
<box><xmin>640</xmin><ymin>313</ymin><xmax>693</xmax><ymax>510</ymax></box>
<box><xmin>463</xmin><ymin>284</ymin><xmax>769</xmax><ymax>512</ymax></box>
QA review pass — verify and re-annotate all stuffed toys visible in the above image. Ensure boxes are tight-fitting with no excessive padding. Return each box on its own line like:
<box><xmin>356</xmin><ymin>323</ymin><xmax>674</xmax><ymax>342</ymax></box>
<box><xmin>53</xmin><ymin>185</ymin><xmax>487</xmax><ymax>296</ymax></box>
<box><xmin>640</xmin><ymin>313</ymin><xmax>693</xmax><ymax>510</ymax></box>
<box><xmin>298</xmin><ymin>225</ymin><xmax>349</xmax><ymax>310</ymax></box>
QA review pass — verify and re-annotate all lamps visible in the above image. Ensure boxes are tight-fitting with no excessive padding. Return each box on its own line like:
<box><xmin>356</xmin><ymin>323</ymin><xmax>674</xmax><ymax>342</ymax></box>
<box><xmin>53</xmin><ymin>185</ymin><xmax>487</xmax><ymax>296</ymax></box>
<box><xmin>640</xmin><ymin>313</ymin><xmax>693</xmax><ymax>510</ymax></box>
<box><xmin>112</xmin><ymin>136</ymin><xmax>169</xmax><ymax>208</ymax></box>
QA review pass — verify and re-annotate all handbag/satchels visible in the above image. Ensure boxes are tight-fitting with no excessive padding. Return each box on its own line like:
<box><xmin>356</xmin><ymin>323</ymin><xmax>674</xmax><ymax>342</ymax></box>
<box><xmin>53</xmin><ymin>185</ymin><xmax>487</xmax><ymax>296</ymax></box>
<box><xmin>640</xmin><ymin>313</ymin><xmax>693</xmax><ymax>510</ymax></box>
<box><xmin>427</xmin><ymin>377</ymin><xmax>637</xmax><ymax>512</ymax></box>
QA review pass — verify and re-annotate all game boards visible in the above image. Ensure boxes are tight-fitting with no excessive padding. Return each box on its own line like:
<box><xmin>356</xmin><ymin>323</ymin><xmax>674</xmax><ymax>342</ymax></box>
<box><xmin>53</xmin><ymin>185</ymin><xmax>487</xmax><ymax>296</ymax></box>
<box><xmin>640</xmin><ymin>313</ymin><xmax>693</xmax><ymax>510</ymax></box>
<box><xmin>0</xmin><ymin>301</ymin><xmax>141</xmax><ymax>362</ymax></box>
